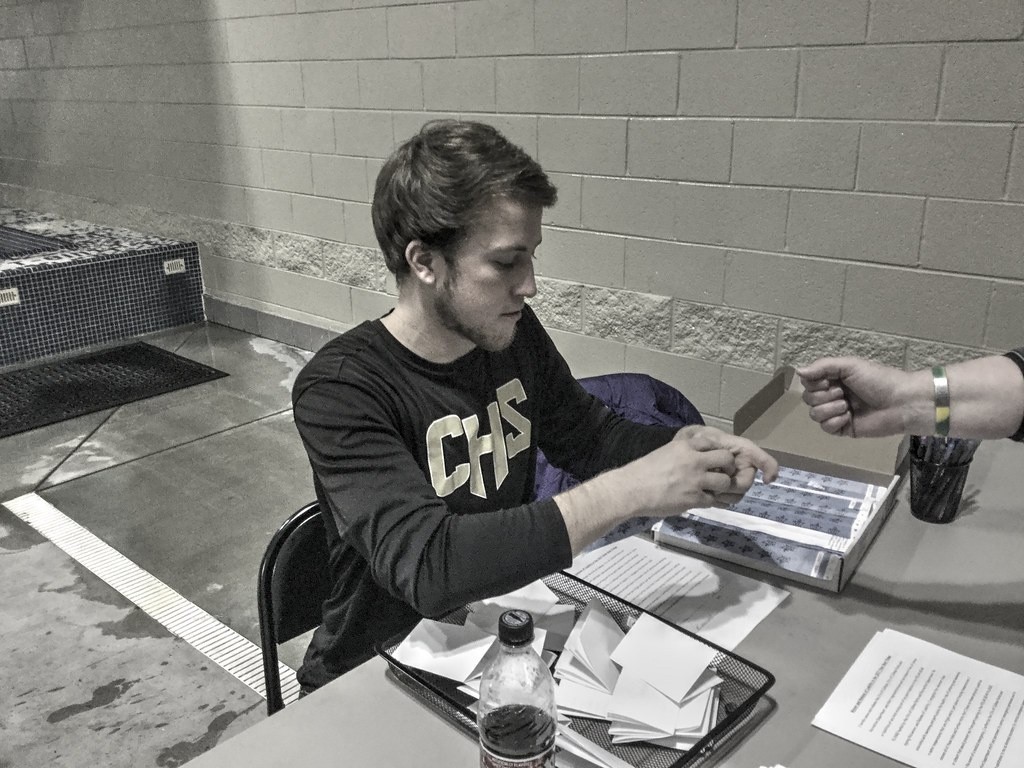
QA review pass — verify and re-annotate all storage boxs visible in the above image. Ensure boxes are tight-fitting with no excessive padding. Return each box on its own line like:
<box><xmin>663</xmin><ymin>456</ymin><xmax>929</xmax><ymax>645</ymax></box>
<box><xmin>652</xmin><ymin>366</ymin><xmax>911</xmax><ymax>594</ymax></box>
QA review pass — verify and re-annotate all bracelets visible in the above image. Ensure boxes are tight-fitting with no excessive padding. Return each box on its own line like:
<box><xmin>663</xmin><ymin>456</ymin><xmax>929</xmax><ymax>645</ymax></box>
<box><xmin>932</xmin><ymin>364</ymin><xmax>951</xmax><ymax>439</ymax></box>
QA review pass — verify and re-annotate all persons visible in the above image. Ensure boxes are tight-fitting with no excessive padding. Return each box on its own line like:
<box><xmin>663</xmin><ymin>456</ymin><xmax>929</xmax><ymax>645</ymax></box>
<box><xmin>796</xmin><ymin>344</ymin><xmax>1024</xmax><ymax>444</ymax></box>
<box><xmin>292</xmin><ymin>117</ymin><xmax>780</xmax><ymax>700</ymax></box>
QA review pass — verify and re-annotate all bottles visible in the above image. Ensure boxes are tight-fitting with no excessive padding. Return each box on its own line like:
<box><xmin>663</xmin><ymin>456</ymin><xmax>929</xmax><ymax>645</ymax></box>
<box><xmin>476</xmin><ymin>611</ymin><xmax>559</xmax><ymax>768</ymax></box>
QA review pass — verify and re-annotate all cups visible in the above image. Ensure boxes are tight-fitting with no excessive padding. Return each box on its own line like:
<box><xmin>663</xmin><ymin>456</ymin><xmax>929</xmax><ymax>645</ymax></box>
<box><xmin>908</xmin><ymin>452</ymin><xmax>973</xmax><ymax>523</ymax></box>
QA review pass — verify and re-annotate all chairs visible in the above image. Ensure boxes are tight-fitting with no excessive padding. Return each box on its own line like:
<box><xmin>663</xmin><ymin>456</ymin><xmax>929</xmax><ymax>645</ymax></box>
<box><xmin>257</xmin><ymin>500</ymin><xmax>332</xmax><ymax>716</ymax></box>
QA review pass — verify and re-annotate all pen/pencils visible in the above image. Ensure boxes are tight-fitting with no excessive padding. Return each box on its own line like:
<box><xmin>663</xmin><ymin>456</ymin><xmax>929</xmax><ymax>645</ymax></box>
<box><xmin>909</xmin><ymin>435</ymin><xmax>983</xmax><ymax>522</ymax></box>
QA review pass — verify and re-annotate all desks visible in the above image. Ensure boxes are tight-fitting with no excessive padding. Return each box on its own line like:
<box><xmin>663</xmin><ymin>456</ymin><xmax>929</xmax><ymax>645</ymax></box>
<box><xmin>175</xmin><ymin>433</ymin><xmax>1024</xmax><ymax>768</ymax></box>
